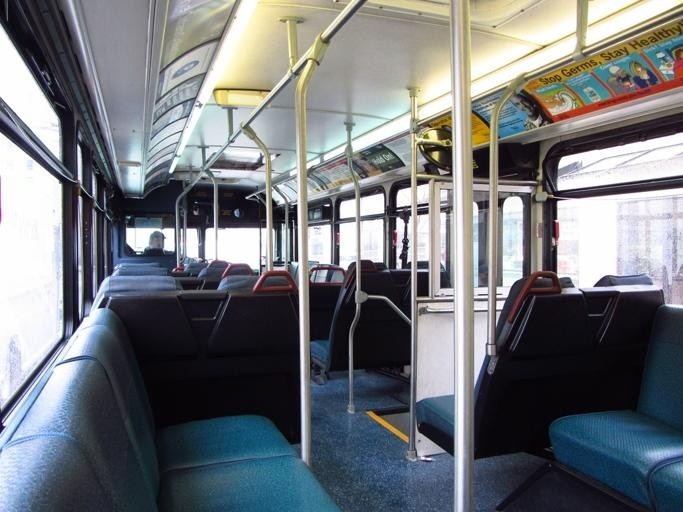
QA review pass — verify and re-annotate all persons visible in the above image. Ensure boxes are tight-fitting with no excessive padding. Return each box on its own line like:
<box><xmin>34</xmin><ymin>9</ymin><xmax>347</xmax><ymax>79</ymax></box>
<box><xmin>143</xmin><ymin>230</ymin><xmax>167</xmax><ymax>256</ymax></box>
<box><xmin>509</xmin><ymin>94</ymin><xmax>549</xmax><ymax>131</ymax></box>
